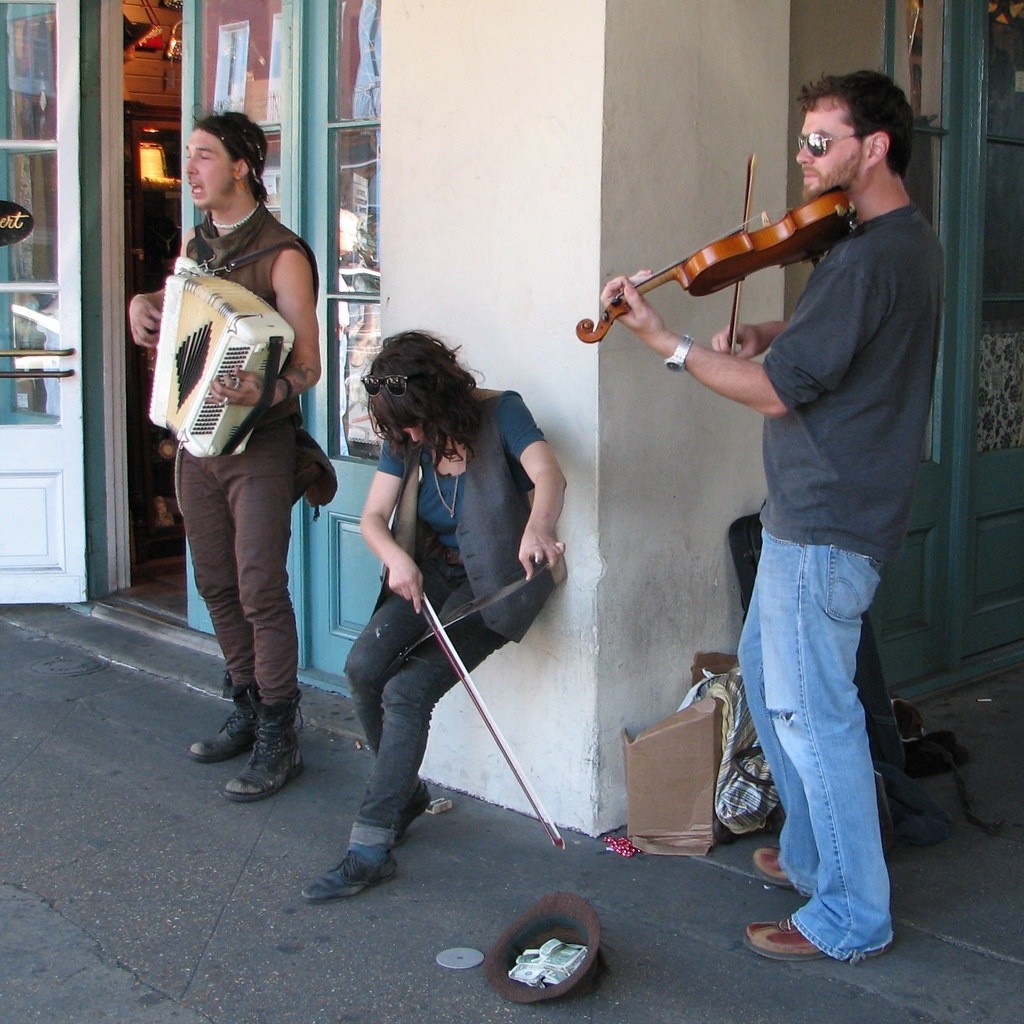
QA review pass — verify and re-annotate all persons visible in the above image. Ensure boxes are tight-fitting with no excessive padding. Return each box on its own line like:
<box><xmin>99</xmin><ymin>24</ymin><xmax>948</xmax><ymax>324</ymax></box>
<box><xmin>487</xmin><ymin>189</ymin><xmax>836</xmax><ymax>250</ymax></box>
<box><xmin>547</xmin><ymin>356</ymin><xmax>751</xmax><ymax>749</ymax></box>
<box><xmin>301</xmin><ymin>330</ymin><xmax>567</xmax><ymax>904</ymax></box>
<box><xmin>128</xmin><ymin>110</ymin><xmax>338</xmax><ymax>803</ymax></box>
<box><xmin>601</xmin><ymin>68</ymin><xmax>947</xmax><ymax>961</ymax></box>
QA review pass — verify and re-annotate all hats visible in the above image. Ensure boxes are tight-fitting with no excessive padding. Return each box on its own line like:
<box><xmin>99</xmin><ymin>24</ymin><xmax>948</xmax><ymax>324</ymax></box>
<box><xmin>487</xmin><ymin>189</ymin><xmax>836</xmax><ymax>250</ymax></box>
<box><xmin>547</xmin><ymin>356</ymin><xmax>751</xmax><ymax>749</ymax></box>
<box><xmin>482</xmin><ymin>893</ymin><xmax>603</xmax><ymax>1004</ymax></box>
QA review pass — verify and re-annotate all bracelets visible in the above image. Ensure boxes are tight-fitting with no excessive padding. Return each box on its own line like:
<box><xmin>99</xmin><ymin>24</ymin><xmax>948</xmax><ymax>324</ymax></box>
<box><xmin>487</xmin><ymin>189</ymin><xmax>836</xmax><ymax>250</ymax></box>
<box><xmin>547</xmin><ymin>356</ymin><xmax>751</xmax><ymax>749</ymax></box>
<box><xmin>281</xmin><ymin>373</ymin><xmax>293</xmax><ymax>401</ymax></box>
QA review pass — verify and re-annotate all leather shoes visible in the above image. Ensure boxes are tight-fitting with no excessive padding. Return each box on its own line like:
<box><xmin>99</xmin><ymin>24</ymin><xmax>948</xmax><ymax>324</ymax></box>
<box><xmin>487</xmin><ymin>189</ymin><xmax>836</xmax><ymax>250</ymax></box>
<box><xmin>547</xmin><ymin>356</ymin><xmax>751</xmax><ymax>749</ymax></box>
<box><xmin>300</xmin><ymin>850</ymin><xmax>399</xmax><ymax>903</ymax></box>
<box><xmin>741</xmin><ymin>917</ymin><xmax>830</xmax><ymax>961</ymax></box>
<box><xmin>393</xmin><ymin>780</ymin><xmax>432</xmax><ymax>846</ymax></box>
<box><xmin>752</xmin><ymin>845</ymin><xmax>793</xmax><ymax>886</ymax></box>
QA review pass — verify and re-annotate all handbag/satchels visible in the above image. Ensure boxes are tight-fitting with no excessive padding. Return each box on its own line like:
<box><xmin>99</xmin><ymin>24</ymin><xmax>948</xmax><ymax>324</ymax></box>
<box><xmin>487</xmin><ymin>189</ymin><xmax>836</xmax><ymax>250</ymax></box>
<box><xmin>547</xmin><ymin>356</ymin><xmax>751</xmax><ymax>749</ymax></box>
<box><xmin>292</xmin><ymin>428</ymin><xmax>337</xmax><ymax>523</ymax></box>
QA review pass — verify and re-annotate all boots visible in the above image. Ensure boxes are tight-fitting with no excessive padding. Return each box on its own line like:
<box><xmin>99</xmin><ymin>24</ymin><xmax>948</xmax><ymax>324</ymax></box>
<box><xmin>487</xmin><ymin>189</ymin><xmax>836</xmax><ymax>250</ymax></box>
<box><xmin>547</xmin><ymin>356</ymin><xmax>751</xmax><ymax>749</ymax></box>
<box><xmin>186</xmin><ymin>672</ymin><xmax>258</xmax><ymax>763</ymax></box>
<box><xmin>222</xmin><ymin>679</ymin><xmax>305</xmax><ymax>801</ymax></box>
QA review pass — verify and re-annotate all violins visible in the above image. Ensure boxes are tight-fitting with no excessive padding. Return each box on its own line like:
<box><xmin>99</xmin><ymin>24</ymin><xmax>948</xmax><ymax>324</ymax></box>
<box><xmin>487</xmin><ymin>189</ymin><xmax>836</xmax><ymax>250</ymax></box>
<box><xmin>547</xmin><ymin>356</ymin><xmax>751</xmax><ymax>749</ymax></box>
<box><xmin>574</xmin><ymin>183</ymin><xmax>851</xmax><ymax>343</ymax></box>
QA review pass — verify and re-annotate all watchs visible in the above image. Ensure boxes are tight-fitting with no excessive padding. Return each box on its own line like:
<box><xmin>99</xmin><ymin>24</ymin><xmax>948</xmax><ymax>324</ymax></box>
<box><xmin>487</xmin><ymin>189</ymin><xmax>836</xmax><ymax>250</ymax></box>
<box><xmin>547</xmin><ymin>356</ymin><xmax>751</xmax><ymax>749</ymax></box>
<box><xmin>664</xmin><ymin>332</ymin><xmax>694</xmax><ymax>373</ymax></box>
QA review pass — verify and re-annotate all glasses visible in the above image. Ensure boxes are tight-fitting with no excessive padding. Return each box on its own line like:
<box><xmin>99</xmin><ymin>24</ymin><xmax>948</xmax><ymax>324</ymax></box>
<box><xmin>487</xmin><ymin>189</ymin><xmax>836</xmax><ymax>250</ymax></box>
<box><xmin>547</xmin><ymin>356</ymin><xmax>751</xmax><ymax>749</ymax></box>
<box><xmin>359</xmin><ymin>373</ymin><xmax>424</xmax><ymax>397</ymax></box>
<box><xmin>796</xmin><ymin>133</ymin><xmax>857</xmax><ymax>159</ymax></box>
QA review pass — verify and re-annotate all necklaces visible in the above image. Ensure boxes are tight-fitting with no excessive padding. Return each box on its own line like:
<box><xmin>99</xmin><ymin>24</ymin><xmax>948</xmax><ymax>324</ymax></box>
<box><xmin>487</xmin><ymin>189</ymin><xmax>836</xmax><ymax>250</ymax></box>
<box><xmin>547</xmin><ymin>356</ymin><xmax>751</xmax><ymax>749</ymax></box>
<box><xmin>427</xmin><ymin>438</ymin><xmax>460</xmax><ymax>518</ymax></box>
<box><xmin>155</xmin><ymin>229</ymin><xmax>178</xmax><ymax>251</ymax></box>
<box><xmin>212</xmin><ymin>201</ymin><xmax>260</xmax><ymax>230</ymax></box>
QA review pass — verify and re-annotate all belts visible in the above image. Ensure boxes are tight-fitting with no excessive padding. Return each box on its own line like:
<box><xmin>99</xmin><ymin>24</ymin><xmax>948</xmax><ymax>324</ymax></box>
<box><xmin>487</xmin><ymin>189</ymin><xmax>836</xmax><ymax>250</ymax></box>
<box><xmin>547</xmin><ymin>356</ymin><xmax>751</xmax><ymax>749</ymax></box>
<box><xmin>444</xmin><ymin>547</ymin><xmax>464</xmax><ymax>565</ymax></box>
